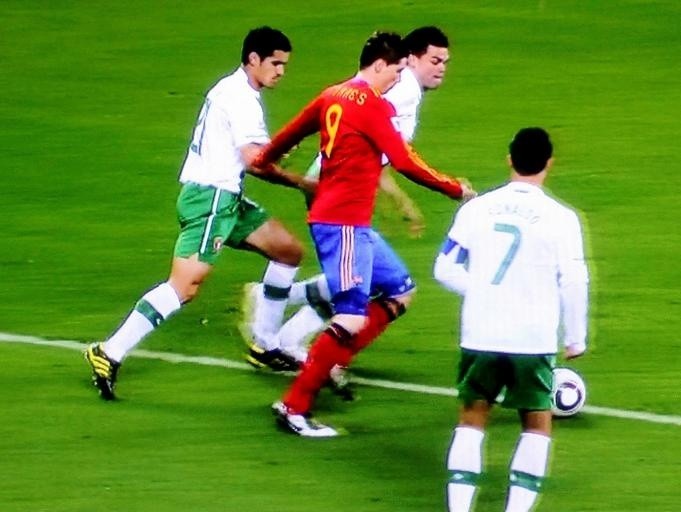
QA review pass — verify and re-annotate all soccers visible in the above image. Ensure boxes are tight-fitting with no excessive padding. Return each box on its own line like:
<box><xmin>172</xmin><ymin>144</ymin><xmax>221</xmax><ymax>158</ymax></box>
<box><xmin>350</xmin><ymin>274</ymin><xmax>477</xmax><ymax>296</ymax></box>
<box><xmin>550</xmin><ymin>369</ymin><xmax>585</xmax><ymax>416</ymax></box>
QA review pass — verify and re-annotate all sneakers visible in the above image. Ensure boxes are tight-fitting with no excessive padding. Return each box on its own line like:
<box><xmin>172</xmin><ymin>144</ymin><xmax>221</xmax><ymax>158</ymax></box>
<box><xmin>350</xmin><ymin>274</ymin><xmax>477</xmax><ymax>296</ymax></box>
<box><xmin>246</xmin><ymin>344</ymin><xmax>297</xmax><ymax>371</ymax></box>
<box><xmin>271</xmin><ymin>400</ymin><xmax>337</xmax><ymax>437</ymax></box>
<box><xmin>82</xmin><ymin>341</ymin><xmax>122</xmax><ymax>400</ymax></box>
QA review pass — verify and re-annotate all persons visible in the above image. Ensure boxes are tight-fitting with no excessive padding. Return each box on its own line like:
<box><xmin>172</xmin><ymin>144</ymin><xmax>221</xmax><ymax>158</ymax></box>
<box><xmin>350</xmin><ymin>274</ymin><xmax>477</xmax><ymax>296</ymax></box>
<box><xmin>251</xmin><ymin>32</ymin><xmax>481</xmax><ymax>441</ymax></box>
<box><xmin>434</xmin><ymin>126</ymin><xmax>592</xmax><ymax>512</ymax></box>
<box><xmin>236</xmin><ymin>23</ymin><xmax>477</xmax><ymax>404</ymax></box>
<box><xmin>84</xmin><ymin>26</ymin><xmax>308</xmax><ymax>403</ymax></box>
